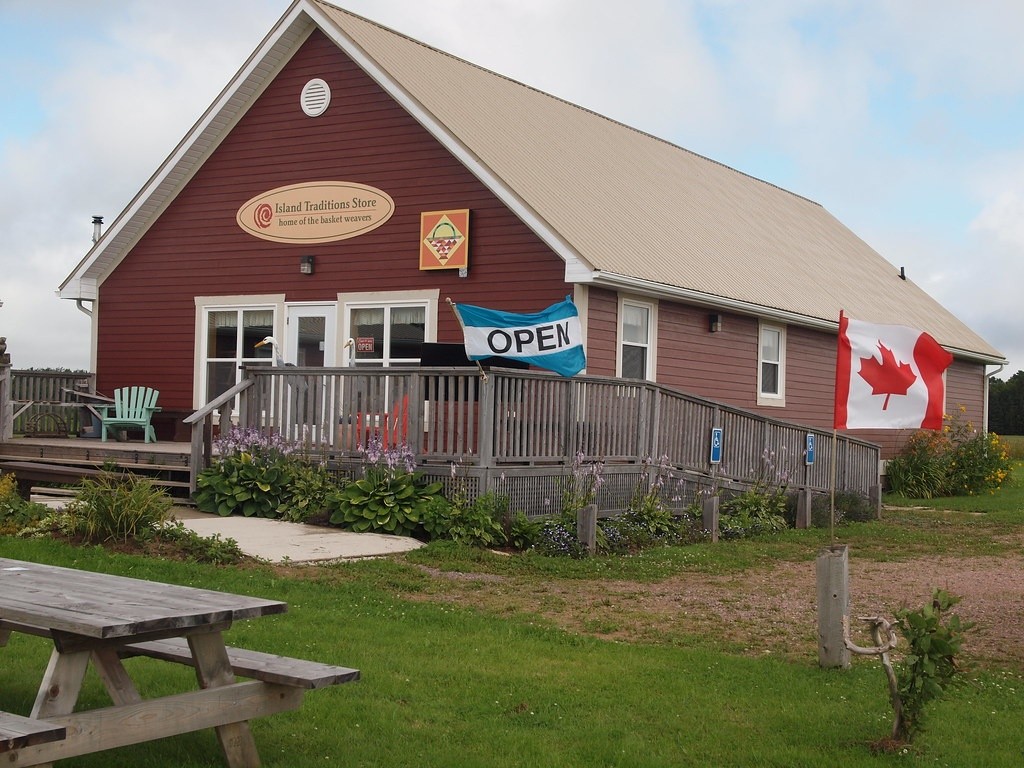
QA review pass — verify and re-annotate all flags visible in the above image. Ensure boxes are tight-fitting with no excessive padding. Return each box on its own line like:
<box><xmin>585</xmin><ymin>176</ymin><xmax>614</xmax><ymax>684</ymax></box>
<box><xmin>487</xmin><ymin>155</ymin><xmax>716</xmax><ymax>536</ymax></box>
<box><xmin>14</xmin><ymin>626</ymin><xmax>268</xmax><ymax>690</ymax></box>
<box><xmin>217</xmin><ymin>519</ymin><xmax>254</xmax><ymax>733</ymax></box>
<box><xmin>835</xmin><ymin>317</ymin><xmax>953</xmax><ymax>429</ymax></box>
<box><xmin>454</xmin><ymin>294</ymin><xmax>585</xmax><ymax>378</ymax></box>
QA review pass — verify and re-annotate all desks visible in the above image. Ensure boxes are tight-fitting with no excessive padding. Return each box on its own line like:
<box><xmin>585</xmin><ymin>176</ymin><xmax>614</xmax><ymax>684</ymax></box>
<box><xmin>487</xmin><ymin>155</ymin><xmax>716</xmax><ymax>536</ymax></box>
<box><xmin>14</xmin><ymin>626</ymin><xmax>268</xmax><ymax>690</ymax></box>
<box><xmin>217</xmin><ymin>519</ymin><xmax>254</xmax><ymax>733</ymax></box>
<box><xmin>0</xmin><ymin>559</ymin><xmax>306</xmax><ymax>768</ymax></box>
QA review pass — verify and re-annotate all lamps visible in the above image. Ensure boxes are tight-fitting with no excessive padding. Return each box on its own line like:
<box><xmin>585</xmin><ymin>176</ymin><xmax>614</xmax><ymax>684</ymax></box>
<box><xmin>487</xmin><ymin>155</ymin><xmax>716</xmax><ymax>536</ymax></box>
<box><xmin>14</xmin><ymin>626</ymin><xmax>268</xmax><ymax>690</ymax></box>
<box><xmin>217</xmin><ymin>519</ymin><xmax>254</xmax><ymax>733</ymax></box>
<box><xmin>708</xmin><ymin>314</ymin><xmax>721</xmax><ymax>332</ymax></box>
<box><xmin>300</xmin><ymin>255</ymin><xmax>314</xmax><ymax>276</ymax></box>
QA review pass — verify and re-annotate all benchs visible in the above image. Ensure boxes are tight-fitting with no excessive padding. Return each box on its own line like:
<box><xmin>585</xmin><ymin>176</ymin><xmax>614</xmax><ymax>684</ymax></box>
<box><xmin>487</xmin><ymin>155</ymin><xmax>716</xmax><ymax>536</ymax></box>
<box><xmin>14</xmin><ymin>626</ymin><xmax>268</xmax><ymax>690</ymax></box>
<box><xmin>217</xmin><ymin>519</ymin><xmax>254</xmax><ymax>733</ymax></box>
<box><xmin>0</xmin><ymin>618</ymin><xmax>361</xmax><ymax>688</ymax></box>
<box><xmin>0</xmin><ymin>710</ymin><xmax>67</xmax><ymax>755</ymax></box>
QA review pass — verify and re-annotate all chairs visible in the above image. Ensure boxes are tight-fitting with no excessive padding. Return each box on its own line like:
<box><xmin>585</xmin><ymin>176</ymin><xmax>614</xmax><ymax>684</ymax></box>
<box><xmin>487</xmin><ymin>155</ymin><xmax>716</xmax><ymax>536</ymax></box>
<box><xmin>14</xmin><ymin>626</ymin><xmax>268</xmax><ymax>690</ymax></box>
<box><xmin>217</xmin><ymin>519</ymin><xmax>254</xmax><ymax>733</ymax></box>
<box><xmin>94</xmin><ymin>386</ymin><xmax>162</xmax><ymax>444</ymax></box>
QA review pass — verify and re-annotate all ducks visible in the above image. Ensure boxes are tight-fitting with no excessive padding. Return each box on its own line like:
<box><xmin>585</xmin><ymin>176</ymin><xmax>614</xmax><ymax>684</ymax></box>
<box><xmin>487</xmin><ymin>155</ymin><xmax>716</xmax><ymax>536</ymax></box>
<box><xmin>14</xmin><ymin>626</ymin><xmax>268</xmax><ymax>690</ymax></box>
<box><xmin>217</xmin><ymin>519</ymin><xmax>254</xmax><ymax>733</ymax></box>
<box><xmin>255</xmin><ymin>336</ymin><xmax>308</xmax><ymax>392</ymax></box>
<box><xmin>344</xmin><ymin>338</ymin><xmax>366</xmax><ymax>393</ymax></box>
